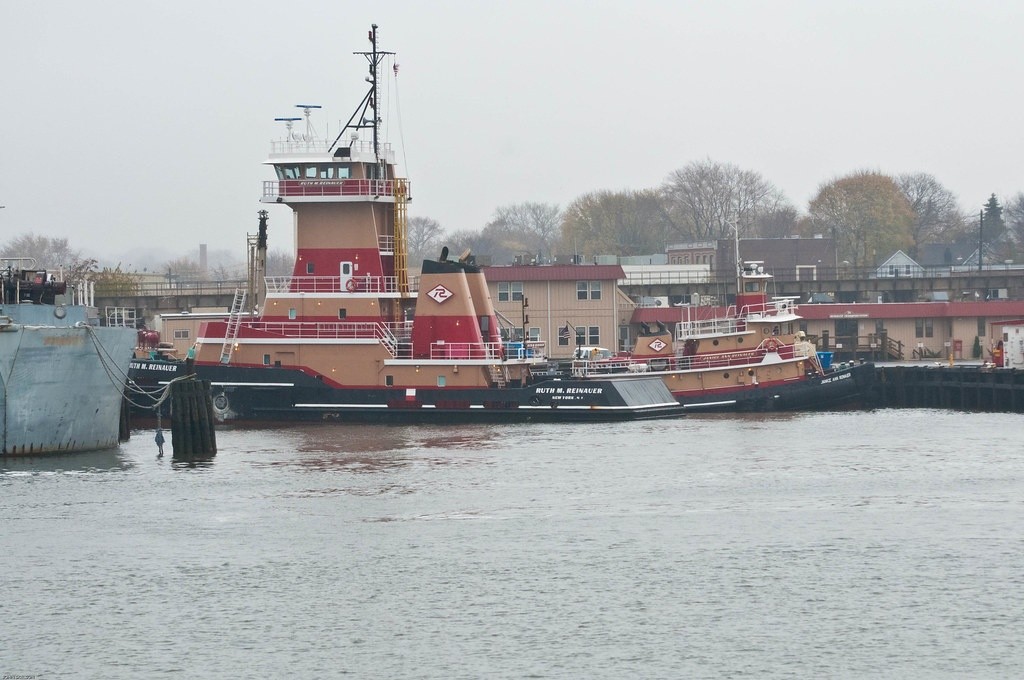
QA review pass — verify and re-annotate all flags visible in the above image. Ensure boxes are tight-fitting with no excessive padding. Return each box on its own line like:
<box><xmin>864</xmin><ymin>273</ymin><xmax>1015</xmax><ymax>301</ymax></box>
<box><xmin>561</xmin><ymin>326</ymin><xmax>571</xmax><ymax>339</ymax></box>
<box><xmin>393</xmin><ymin>64</ymin><xmax>399</xmax><ymax>76</ymax></box>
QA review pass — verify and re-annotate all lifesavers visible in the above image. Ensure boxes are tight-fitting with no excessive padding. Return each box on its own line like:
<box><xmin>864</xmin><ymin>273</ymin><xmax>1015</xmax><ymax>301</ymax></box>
<box><xmin>765</xmin><ymin>338</ymin><xmax>777</xmax><ymax>352</ymax></box>
<box><xmin>345</xmin><ymin>278</ymin><xmax>358</xmax><ymax>292</ymax></box>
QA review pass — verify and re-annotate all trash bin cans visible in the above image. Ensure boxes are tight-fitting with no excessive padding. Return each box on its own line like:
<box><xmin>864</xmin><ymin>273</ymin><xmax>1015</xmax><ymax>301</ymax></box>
<box><xmin>816</xmin><ymin>351</ymin><xmax>834</xmax><ymax>370</ymax></box>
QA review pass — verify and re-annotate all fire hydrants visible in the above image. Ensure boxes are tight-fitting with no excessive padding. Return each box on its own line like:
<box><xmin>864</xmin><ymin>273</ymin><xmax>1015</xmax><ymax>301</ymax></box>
<box><xmin>949</xmin><ymin>354</ymin><xmax>954</xmax><ymax>366</ymax></box>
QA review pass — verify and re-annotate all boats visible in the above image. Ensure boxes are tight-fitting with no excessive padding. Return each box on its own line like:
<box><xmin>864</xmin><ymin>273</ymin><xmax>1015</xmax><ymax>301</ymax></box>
<box><xmin>125</xmin><ymin>24</ymin><xmax>690</xmax><ymax>424</ymax></box>
<box><xmin>563</xmin><ymin>213</ymin><xmax>878</xmax><ymax>418</ymax></box>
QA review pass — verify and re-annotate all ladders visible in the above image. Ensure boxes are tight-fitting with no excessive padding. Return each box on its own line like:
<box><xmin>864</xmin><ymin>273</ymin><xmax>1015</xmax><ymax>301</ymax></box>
<box><xmin>219</xmin><ymin>288</ymin><xmax>247</xmax><ymax>364</ymax></box>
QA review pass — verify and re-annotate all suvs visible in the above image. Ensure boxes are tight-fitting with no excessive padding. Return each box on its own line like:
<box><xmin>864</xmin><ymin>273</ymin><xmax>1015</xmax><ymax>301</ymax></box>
<box><xmin>571</xmin><ymin>347</ymin><xmax>612</xmax><ymax>360</ymax></box>
